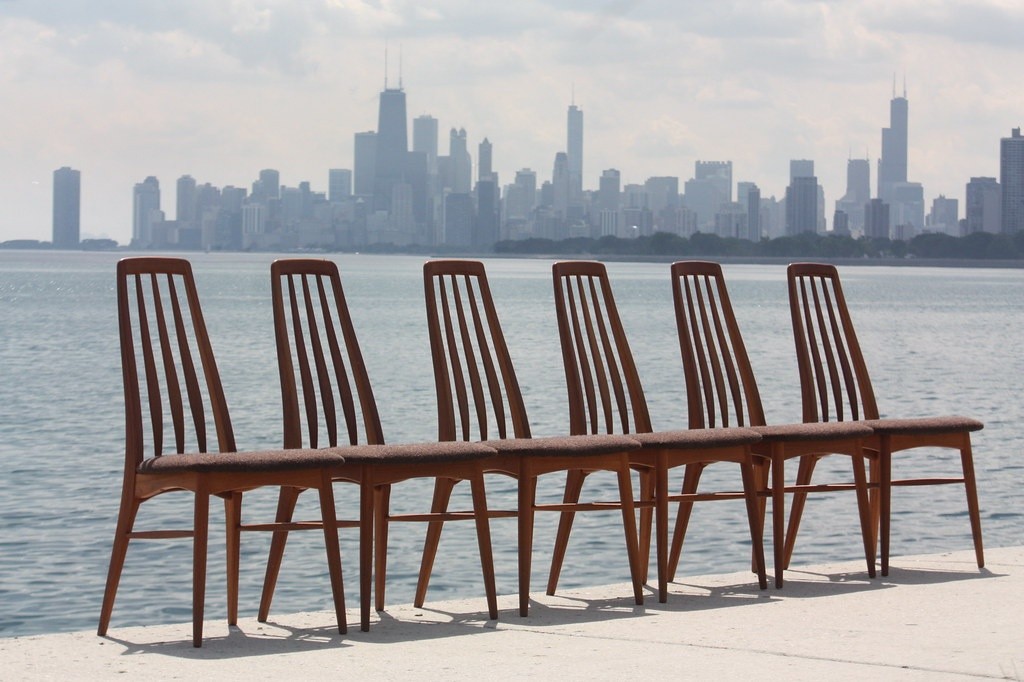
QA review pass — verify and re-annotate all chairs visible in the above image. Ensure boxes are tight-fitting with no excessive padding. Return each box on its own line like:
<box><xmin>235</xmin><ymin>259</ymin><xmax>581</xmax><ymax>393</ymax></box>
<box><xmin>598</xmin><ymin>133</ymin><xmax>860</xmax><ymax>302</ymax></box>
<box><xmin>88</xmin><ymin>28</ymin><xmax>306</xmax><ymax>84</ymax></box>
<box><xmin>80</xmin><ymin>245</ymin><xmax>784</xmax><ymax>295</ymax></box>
<box><xmin>667</xmin><ymin>261</ymin><xmax>878</xmax><ymax>590</ymax></box>
<box><xmin>257</xmin><ymin>259</ymin><xmax>502</xmax><ymax>635</ymax></box>
<box><xmin>413</xmin><ymin>261</ymin><xmax>645</xmax><ymax>620</ymax></box>
<box><xmin>783</xmin><ymin>264</ymin><xmax>985</xmax><ymax>578</ymax></box>
<box><xmin>97</xmin><ymin>257</ymin><xmax>347</xmax><ymax>648</ymax></box>
<box><xmin>546</xmin><ymin>260</ymin><xmax>768</xmax><ymax>605</ymax></box>
